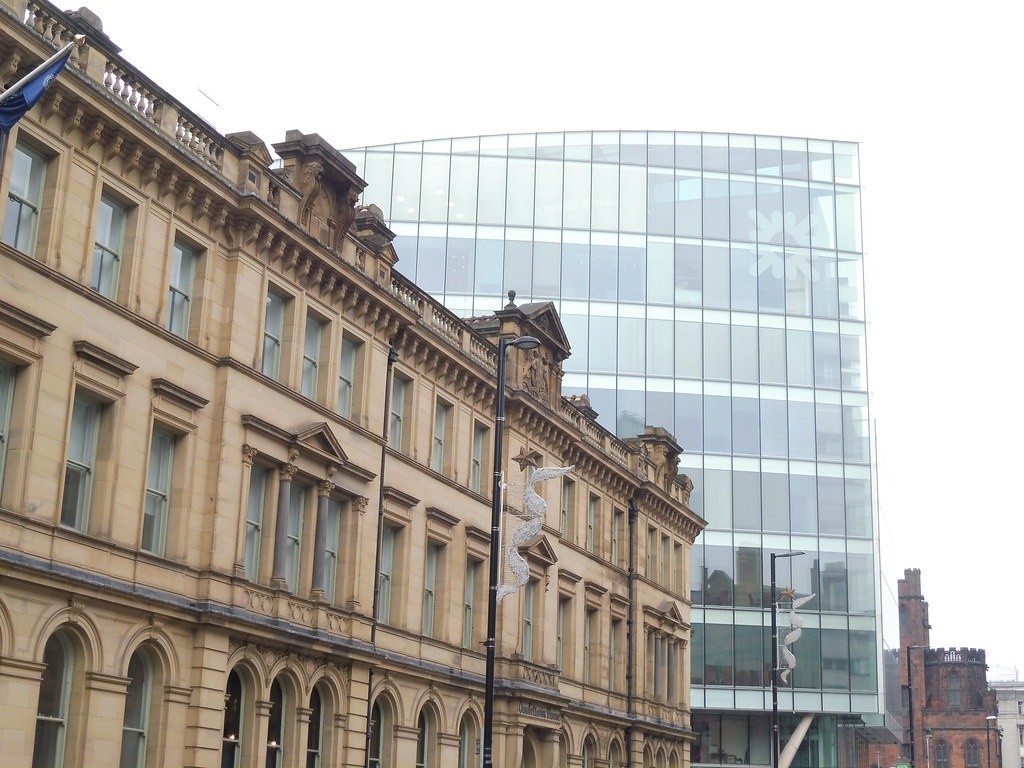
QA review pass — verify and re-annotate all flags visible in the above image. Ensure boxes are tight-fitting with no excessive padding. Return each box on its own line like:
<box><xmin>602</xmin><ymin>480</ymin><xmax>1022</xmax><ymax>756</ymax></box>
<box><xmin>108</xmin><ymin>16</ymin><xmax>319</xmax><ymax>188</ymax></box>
<box><xmin>0</xmin><ymin>49</ymin><xmax>71</xmax><ymax>135</ymax></box>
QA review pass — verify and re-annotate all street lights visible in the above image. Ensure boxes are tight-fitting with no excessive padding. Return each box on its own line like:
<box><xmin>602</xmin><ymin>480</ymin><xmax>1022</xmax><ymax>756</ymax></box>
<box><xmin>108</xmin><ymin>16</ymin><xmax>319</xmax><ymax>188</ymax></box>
<box><xmin>482</xmin><ymin>335</ymin><xmax>543</xmax><ymax>768</ymax></box>
<box><xmin>987</xmin><ymin>716</ymin><xmax>998</xmax><ymax>768</ymax></box>
<box><xmin>770</xmin><ymin>550</ymin><xmax>806</xmax><ymax>768</ymax></box>
<box><xmin>906</xmin><ymin>645</ymin><xmax>931</xmax><ymax>768</ymax></box>
